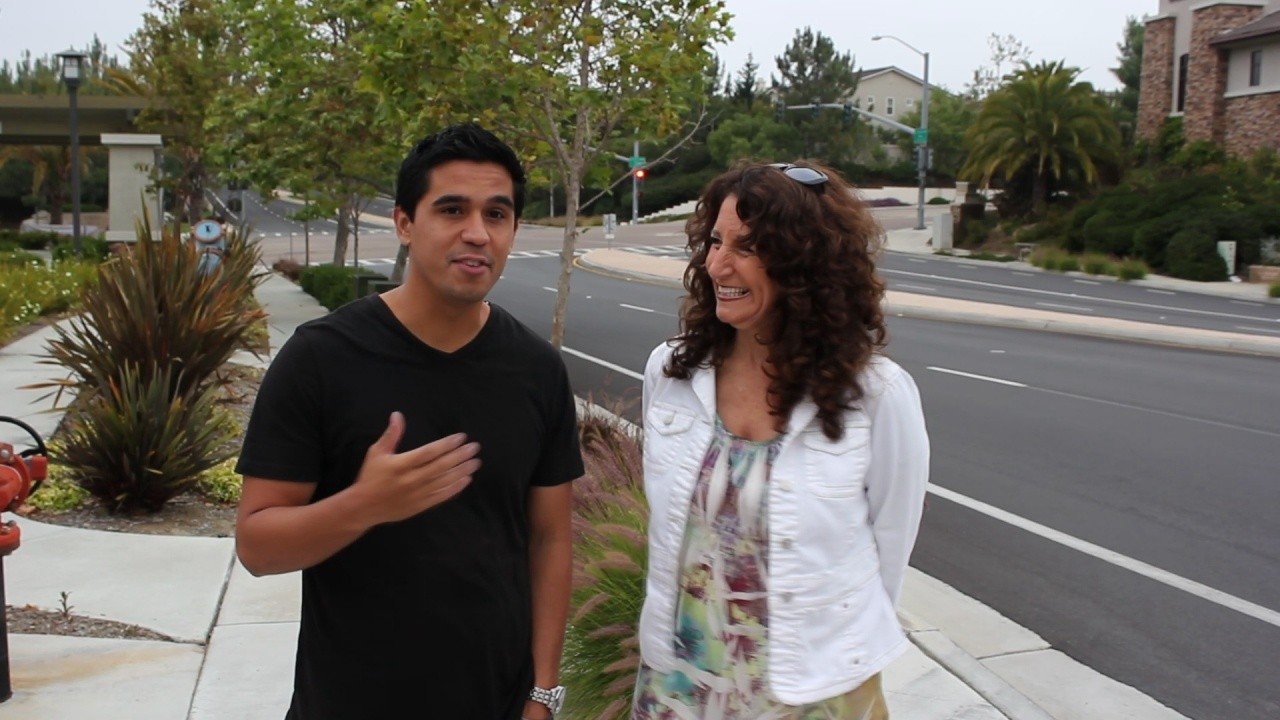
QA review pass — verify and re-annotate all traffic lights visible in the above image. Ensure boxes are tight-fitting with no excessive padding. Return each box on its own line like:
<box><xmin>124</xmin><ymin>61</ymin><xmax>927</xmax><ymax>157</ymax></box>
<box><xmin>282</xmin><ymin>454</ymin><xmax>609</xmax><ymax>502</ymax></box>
<box><xmin>843</xmin><ymin>103</ymin><xmax>852</xmax><ymax>123</ymax></box>
<box><xmin>776</xmin><ymin>99</ymin><xmax>785</xmax><ymax>119</ymax></box>
<box><xmin>811</xmin><ymin>100</ymin><xmax>821</xmax><ymax>118</ymax></box>
<box><xmin>633</xmin><ymin>168</ymin><xmax>647</xmax><ymax>179</ymax></box>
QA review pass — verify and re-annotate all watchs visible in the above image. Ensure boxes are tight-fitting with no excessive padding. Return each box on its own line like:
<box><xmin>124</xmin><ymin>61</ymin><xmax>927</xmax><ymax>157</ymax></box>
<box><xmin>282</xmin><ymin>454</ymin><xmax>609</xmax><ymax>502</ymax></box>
<box><xmin>528</xmin><ymin>686</ymin><xmax>566</xmax><ymax>714</ymax></box>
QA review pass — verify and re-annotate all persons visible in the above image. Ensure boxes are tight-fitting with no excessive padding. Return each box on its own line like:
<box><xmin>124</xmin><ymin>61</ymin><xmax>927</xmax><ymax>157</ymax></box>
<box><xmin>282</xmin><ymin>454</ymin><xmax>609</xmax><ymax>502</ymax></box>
<box><xmin>234</xmin><ymin>122</ymin><xmax>585</xmax><ymax>720</ymax></box>
<box><xmin>632</xmin><ymin>163</ymin><xmax>930</xmax><ymax>720</ymax></box>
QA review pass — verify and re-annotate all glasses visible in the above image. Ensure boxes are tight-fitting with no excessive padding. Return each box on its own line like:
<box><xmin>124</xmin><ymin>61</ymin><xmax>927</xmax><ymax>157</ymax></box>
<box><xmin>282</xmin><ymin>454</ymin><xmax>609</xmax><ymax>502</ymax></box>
<box><xmin>767</xmin><ymin>162</ymin><xmax>828</xmax><ymax>185</ymax></box>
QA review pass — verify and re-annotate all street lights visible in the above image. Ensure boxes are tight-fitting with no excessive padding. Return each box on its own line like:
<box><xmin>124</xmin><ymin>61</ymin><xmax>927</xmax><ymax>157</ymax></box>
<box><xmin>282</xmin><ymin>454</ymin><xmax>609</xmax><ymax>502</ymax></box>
<box><xmin>53</xmin><ymin>44</ymin><xmax>90</xmax><ymax>251</ymax></box>
<box><xmin>872</xmin><ymin>34</ymin><xmax>931</xmax><ymax>230</ymax></box>
